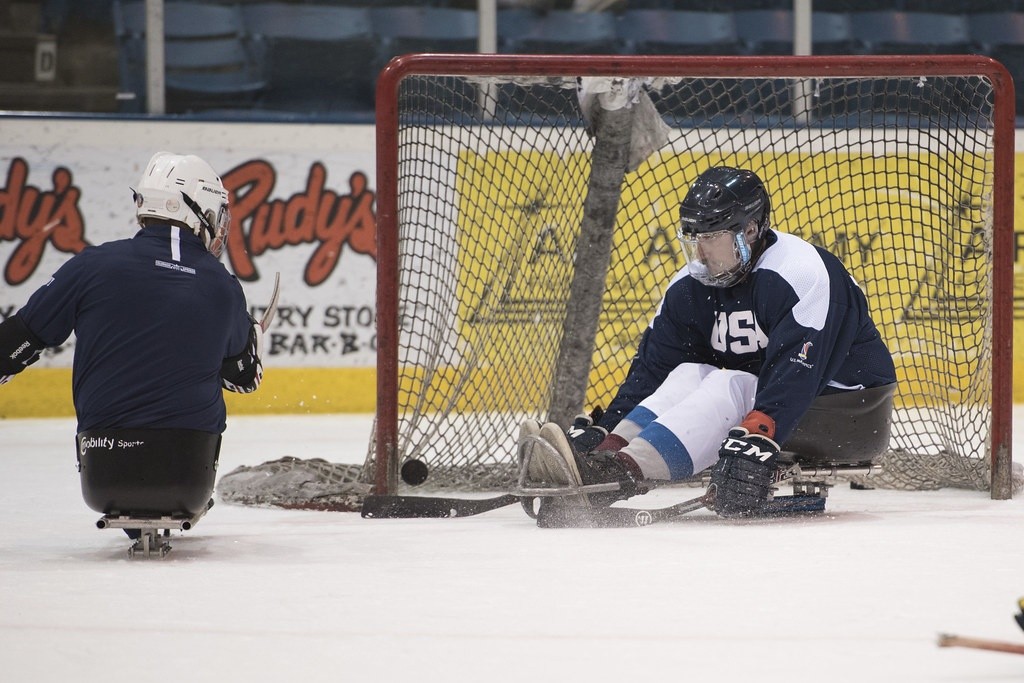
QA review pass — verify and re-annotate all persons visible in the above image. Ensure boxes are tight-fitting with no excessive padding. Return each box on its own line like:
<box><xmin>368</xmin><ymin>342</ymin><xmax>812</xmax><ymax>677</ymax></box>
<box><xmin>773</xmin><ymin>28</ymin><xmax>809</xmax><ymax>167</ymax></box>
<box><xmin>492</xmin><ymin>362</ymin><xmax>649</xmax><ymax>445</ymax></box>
<box><xmin>518</xmin><ymin>166</ymin><xmax>897</xmax><ymax>515</ymax></box>
<box><xmin>0</xmin><ymin>151</ymin><xmax>262</xmax><ymax>515</ymax></box>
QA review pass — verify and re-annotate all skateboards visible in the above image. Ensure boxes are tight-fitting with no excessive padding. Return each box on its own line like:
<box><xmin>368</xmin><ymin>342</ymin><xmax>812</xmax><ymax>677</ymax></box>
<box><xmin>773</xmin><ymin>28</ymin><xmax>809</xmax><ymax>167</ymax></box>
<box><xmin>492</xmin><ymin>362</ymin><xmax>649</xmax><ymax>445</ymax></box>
<box><xmin>96</xmin><ymin>498</ymin><xmax>214</xmax><ymax>561</ymax></box>
<box><xmin>511</xmin><ymin>436</ymin><xmax>882</xmax><ymax>510</ymax></box>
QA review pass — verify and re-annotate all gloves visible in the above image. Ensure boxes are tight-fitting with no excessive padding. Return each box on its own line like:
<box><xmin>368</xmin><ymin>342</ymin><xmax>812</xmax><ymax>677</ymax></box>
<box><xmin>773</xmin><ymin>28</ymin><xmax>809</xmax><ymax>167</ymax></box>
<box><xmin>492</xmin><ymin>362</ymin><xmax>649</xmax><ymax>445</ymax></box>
<box><xmin>705</xmin><ymin>428</ymin><xmax>781</xmax><ymax>515</ymax></box>
<box><xmin>566</xmin><ymin>405</ymin><xmax>606</xmax><ymax>439</ymax></box>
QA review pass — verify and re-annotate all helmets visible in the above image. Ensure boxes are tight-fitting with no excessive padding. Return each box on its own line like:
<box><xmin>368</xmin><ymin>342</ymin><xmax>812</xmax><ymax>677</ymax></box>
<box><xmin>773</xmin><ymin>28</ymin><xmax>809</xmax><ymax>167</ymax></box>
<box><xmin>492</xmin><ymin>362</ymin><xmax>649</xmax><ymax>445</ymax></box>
<box><xmin>679</xmin><ymin>166</ymin><xmax>771</xmax><ymax>288</ymax></box>
<box><xmin>136</xmin><ymin>151</ymin><xmax>230</xmax><ymax>256</ymax></box>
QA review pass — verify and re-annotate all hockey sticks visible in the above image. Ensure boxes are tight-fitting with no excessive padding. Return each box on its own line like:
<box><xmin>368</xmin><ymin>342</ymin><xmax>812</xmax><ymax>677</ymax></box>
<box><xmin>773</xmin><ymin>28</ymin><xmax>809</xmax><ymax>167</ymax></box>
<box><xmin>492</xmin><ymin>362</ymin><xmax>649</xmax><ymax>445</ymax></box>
<box><xmin>260</xmin><ymin>270</ymin><xmax>281</xmax><ymax>333</ymax></box>
<box><xmin>536</xmin><ymin>495</ymin><xmax>710</xmax><ymax>527</ymax></box>
<box><xmin>360</xmin><ymin>492</ymin><xmax>529</xmax><ymax>518</ymax></box>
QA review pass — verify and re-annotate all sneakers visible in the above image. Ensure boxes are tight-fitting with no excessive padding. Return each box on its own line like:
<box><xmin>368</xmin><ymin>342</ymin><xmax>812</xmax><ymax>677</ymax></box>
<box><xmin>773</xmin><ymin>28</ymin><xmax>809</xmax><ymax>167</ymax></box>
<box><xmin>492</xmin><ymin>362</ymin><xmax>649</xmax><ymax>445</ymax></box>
<box><xmin>517</xmin><ymin>416</ymin><xmax>608</xmax><ymax>486</ymax></box>
<box><xmin>539</xmin><ymin>422</ymin><xmax>648</xmax><ymax>510</ymax></box>
